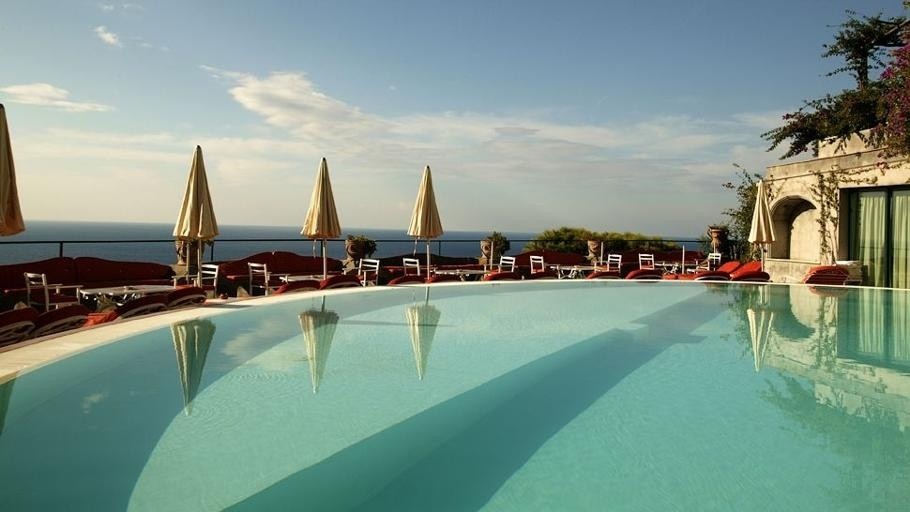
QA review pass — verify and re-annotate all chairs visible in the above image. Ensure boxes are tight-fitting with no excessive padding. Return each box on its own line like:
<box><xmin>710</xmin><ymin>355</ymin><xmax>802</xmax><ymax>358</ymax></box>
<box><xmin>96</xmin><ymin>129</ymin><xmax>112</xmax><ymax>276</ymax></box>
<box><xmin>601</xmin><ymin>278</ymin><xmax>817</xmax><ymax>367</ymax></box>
<box><xmin>800</xmin><ymin>265</ymin><xmax>849</xmax><ymax>286</ymax></box>
<box><xmin>248</xmin><ymin>258</ymin><xmax>380</xmax><ymax>297</ymax></box>
<box><xmin>171</xmin><ymin>264</ymin><xmax>219</xmax><ymax>299</ymax></box>
<box><xmin>388</xmin><ymin>253</ymin><xmax>770</xmax><ymax>286</ymax></box>
<box><xmin>0</xmin><ymin>286</ymin><xmax>207</xmax><ymax>347</ymax></box>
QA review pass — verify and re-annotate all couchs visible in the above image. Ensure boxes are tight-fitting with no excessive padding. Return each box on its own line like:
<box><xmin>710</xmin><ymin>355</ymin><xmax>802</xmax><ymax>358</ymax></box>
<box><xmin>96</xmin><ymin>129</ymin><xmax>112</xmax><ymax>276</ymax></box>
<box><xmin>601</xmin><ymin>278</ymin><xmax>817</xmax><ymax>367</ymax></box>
<box><xmin>220</xmin><ymin>251</ymin><xmax>340</xmax><ymax>287</ymax></box>
<box><xmin>0</xmin><ymin>256</ymin><xmax>176</xmax><ymax>313</ymax></box>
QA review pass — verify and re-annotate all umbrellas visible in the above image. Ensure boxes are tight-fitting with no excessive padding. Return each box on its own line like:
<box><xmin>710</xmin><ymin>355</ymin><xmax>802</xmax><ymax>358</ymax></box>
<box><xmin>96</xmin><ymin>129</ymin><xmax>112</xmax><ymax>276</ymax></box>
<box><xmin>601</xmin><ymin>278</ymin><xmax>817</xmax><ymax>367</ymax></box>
<box><xmin>1</xmin><ymin>101</ymin><xmax>24</xmax><ymax>239</ymax></box>
<box><xmin>300</xmin><ymin>157</ymin><xmax>345</xmax><ymax>279</ymax></box>
<box><xmin>0</xmin><ymin>373</ymin><xmax>24</xmax><ymax>439</ymax></box>
<box><xmin>405</xmin><ymin>167</ymin><xmax>444</xmax><ymax>278</ymax></box>
<box><xmin>171</xmin><ymin>319</ymin><xmax>217</xmax><ymax>419</ymax></box>
<box><xmin>745</xmin><ymin>179</ymin><xmax>778</xmax><ymax>273</ymax></box>
<box><xmin>170</xmin><ymin>144</ymin><xmax>220</xmax><ymax>289</ymax></box>
<box><xmin>744</xmin><ymin>285</ymin><xmax>776</xmax><ymax>375</ymax></box>
<box><xmin>295</xmin><ymin>295</ymin><xmax>341</xmax><ymax>394</ymax></box>
<box><xmin>404</xmin><ymin>286</ymin><xmax>444</xmax><ymax>383</ymax></box>
